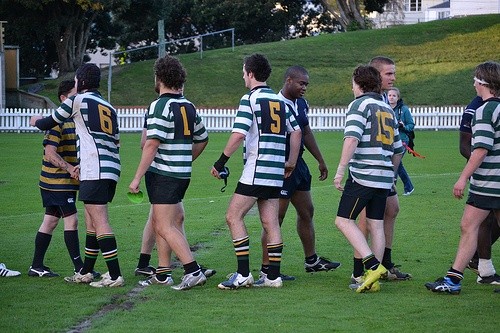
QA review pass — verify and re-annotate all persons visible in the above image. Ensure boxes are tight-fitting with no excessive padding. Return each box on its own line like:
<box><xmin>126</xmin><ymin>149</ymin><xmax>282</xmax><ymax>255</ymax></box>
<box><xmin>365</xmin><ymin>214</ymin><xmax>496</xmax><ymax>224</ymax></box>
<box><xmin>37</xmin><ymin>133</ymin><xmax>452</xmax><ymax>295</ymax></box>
<box><xmin>0</xmin><ymin>262</ymin><xmax>21</xmax><ymax>277</ymax></box>
<box><xmin>334</xmin><ymin>64</ymin><xmax>404</xmax><ymax>293</ymax></box>
<box><xmin>28</xmin><ymin>77</ymin><xmax>100</xmax><ymax>280</ymax></box>
<box><xmin>258</xmin><ymin>65</ymin><xmax>341</xmax><ymax>280</ymax></box>
<box><xmin>425</xmin><ymin>59</ymin><xmax>500</xmax><ymax>295</ymax></box>
<box><xmin>30</xmin><ymin>62</ymin><xmax>126</xmax><ymax>287</ymax></box>
<box><xmin>386</xmin><ymin>86</ymin><xmax>414</xmax><ymax>195</ymax></box>
<box><xmin>130</xmin><ymin>53</ymin><xmax>209</xmax><ymax>290</ymax></box>
<box><xmin>210</xmin><ymin>52</ymin><xmax>302</xmax><ymax>290</ymax></box>
<box><xmin>347</xmin><ymin>56</ymin><xmax>415</xmax><ymax>288</ymax></box>
<box><xmin>459</xmin><ymin>95</ymin><xmax>500</xmax><ymax>284</ymax></box>
<box><xmin>135</xmin><ymin>82</ymin><xmax>216</xmax><ymax>279</ymax></box>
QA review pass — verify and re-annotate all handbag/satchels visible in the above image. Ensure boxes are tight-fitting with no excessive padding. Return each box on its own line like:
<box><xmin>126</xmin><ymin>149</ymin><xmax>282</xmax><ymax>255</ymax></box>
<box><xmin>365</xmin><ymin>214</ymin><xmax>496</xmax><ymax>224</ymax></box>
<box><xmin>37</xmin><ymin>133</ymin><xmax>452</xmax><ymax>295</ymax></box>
<box><xmin>408</xmin><ymin>130</ymin><xmax>415</xmax><ymax>155</ymax></box>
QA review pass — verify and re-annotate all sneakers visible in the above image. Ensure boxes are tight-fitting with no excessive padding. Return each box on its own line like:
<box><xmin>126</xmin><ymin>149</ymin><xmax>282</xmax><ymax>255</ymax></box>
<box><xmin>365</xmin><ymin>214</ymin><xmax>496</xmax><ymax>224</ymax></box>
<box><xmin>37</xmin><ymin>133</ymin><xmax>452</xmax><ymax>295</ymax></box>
<box><xmin>91</xmin><ymin>271</ymin><xmax>100</xmax><ymax>279</ymax></box>
<box><xmin>477</xmin><ymin>273</ymin><xmax>500</xmax><ymax>285</ymax></box>
<box><xmin>183</xmin><ymin>267</ymin><xmax>216</xmax><ymax>277</ymax></box>
<box><xmin>170</xmin><ymin>271</ymin><xmax>207</xmax><ymax>290</ymax></box>
<box><xmin>218</xmin><ymin>271</ymin><xmax>254</xmax><ymax>289</ymax></box>
<box><xmin>0</xmin><ymin>262</ymin><xmax>21</xmax><ymax>277</ymax></box>
<box><xmin>425</xmin><ymin>276</ymin><xmax>462</xmax><ymax>294</ymax></box>
<box><xmin>27</xmin><ymin>265</ymin><xmax>60</xmax><ymax>277</ymax></box>
<box><xmin>349</xmin><ymin>263</ymin><xmax>387</xmax><ymax>293</ymax></box>
<box><xmin>63</xmin><ymin>268</ymin><xmax>94</xmax><ymax>283</ymax></box>
<box><xmin>139</xmin><ymin>274</ymin><xmax>174</xmax><ymax>286</ymax></box>
<box><xmin>89</xmin><ymin>271</ymin><xmax>125</xmax><ymax>287</ymax></box>
<box><xmin>252</xmin><ymin>274</ymin><xmax>283</xmax><ymax>287</ymax></box>
<box><xmin>380</xmin><ymin>266</ymin><xmax>412</xmax><ymax>280</ymax></box>
<box><xmin>135</xmin><ymin>265</ymin><xmax>157</xmax><ymax>276</ymax></box>
<box><xmin>466</xmin><ymin>260</ymin><xmax>478</xmax><ymax>271</ymax></box>
<box><xmin>304</xmin><ymin>256</ymin><xmax>341</xmax><ymax>272</ymax></box>
<box><xmin>258</xmin><ymin>270</ymin><xmax>295</xmax><ymax>280</ymax></box>
<box><xmin>350</xmin><ymin>273</ymin><xmax>365</xmax><ymax>284</ymax></box>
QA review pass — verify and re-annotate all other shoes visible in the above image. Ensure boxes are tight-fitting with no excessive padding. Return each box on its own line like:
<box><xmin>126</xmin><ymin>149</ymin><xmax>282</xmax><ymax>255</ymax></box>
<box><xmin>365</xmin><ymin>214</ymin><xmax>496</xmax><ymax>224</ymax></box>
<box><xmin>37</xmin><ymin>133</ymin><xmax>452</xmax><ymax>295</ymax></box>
<box><xmin>402</xmin><ymin>187</ymin><xmax>415</xmax><ymax>195</ymax></box>
